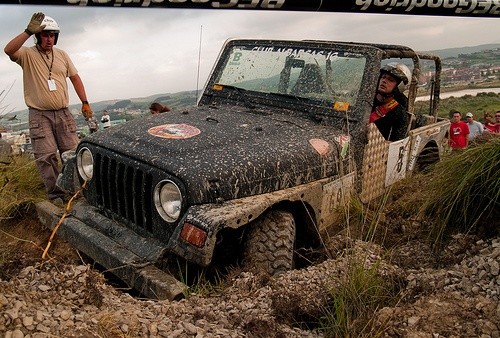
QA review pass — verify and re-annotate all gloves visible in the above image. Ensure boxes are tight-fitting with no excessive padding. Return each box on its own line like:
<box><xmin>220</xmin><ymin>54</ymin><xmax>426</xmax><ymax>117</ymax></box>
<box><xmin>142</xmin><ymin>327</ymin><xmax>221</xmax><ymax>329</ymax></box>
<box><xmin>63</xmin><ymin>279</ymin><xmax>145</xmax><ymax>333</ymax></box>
<box><xmin>25</xmin><ymin>13</ymin><xmax>47</xmax><ymax>36</ymax></box>
<box><xmin>81</xmin><ymin>101</ymin><xmax>93</xmax><ymax>121</ymax></box>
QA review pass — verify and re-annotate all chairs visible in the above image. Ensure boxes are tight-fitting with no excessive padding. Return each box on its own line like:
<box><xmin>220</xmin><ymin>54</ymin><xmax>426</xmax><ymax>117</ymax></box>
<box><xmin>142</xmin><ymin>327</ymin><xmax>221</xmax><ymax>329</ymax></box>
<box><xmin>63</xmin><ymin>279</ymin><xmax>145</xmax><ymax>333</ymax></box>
<box><xmin>290</xmin><ymin>64</ymin><xmax>330</xmax><ymax>95</ymax></box>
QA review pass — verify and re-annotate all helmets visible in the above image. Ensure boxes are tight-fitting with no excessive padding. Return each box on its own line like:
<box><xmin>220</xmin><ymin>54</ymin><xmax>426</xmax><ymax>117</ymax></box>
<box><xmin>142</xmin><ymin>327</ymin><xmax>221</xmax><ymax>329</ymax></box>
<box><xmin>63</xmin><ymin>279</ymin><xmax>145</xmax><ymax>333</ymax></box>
<box><xmin>379</xmin><ymin>61</ymin><xmax>412</xmax><ymax>94</ymax></box>
<box><xmin>32</xmin><ymin>16</ymin><xmax>59</xmax><ymax>45</ymax></box>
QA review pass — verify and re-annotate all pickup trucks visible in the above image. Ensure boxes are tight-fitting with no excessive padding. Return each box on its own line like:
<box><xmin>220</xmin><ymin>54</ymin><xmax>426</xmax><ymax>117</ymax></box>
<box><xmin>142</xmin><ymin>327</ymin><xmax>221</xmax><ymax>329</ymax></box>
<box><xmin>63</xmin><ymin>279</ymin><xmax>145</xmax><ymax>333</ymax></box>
<box><xmin>34</xmin><ymin>36</ymin><xmax>452</xmax><ymax>304</ymax></box>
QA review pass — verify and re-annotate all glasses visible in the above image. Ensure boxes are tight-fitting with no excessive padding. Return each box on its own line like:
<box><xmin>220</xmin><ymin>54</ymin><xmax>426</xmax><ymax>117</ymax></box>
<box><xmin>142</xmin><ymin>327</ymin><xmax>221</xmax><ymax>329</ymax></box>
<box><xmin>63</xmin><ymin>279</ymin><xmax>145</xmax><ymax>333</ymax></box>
<box><xmin>495</xmin><ymin>115</ymin><xmax>500</xmax><ymax>117</ymax></box>
<box><xmin>466</xmin><ymin>116</ymin><xmax>471</xmax><ymax>119</ymax></box>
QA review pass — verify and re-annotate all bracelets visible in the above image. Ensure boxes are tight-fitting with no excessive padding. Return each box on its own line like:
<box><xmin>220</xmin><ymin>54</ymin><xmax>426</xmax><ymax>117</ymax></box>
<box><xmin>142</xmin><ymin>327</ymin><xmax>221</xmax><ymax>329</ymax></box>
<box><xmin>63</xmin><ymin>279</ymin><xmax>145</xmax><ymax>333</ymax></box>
<box><xmin>82</xmin><ymin>100</ymin><xmax>88</xmax><ymax>105</ymax></box>
<box><xmin>24</xmin><ymin>28</ymin><xmax>33</xmax><ymax>37</ymax></box>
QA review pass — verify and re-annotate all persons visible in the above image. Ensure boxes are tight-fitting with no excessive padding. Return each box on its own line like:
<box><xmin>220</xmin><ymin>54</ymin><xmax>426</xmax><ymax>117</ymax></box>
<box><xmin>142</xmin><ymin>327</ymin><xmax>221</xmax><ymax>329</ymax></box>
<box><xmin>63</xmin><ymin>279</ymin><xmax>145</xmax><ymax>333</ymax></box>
<box><xmin>449</xmin><ymin>108</ymin><xmax>500</xmax><ymax>156</ymax></box>
<box><xmin>87</xmin><ymin>112</ymin><xmax>99</xmax><ymax>134</ymax></box>
<box><xmin>100</xmin><ymin>110</ymin><xmax>112</xmax><ymax>129</ymax></box>
<box><xmin>3</xmin><ymin>12</ymin><xmax>94</xmax><ymax>205</ymax></box>
<box><xmin>369</xmin><ymin>61</ymin><xmax>411</xmax><ymax>142</ymax></box>
<box><xmin>0</xmin><ymin>133</ymin><xmax>13</xmax><ymax>165</ymax></box>
<box><xmin>149</xmin><ymin>102</ymin><xmax>172</xmax><ymax>115</ymax></box>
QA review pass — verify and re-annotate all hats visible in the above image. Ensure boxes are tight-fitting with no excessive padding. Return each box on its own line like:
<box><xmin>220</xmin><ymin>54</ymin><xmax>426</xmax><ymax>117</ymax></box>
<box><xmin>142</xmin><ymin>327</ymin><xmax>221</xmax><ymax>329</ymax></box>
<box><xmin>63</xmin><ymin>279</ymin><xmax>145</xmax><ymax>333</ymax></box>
<box><xmin>466</xmin><ymin>112</ymin><xmax>473</xmax><ymax>117</ymax></box>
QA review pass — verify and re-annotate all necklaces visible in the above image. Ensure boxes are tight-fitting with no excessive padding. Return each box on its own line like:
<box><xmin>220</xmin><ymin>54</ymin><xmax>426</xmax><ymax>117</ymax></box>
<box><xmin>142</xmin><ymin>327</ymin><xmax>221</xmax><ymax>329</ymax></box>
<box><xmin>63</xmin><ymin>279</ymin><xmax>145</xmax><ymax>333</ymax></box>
<box><xmin>36</xmin><ymin>45</ymin><xmax>52</xmax><ymax>59</ymax></box>
